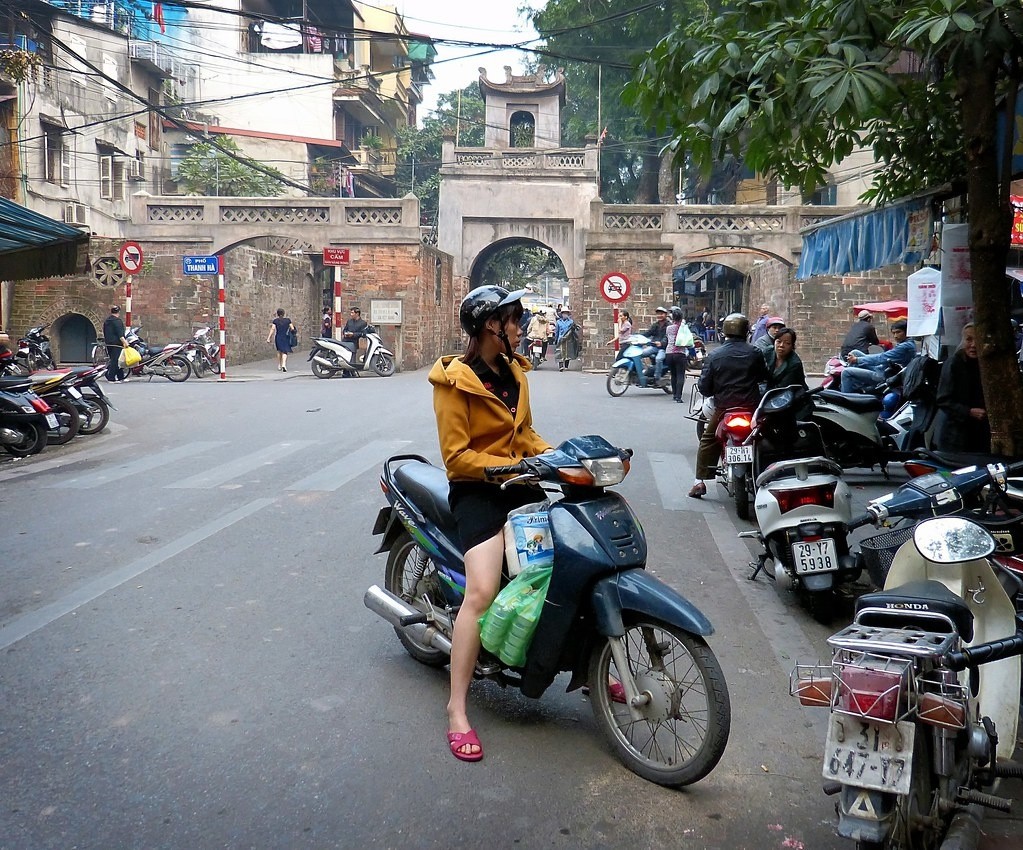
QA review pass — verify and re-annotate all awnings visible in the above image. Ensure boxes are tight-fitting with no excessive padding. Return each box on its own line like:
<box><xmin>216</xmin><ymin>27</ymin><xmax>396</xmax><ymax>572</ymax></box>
<box><xmin>0</xmin><ymin>197</ymin><xmax>92</xmax><ymax>283</ymax></box>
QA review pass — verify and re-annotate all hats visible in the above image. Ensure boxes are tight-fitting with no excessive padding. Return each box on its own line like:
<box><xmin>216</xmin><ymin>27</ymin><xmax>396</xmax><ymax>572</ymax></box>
<box><xmin>858</xmin><ymin>310</ymin><xmax>872</xmax><ymax>319</ymax></box>
<box><xmin>558</xmin><ymin>306</ymin><xmax>570</xmax><ymax>313</ymax></box>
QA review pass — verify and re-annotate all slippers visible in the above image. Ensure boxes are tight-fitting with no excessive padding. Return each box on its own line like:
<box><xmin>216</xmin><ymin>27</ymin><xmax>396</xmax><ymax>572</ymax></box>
<box><xmin>582</xmin><ymin>683</ymin><xmax>626</xmax><ymax>704</ymax></box>
<box><xmin>447</xmin><ymin>727</ymin><xmax>483</xmax><ymax>762</ymax></box>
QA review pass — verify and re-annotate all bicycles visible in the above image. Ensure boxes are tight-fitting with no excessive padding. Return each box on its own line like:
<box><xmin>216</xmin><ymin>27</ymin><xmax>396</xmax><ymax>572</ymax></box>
<box><xmin>92</xmin><ymin>331</ymin><xmax>131</xmax><ymax>382</ymax></box>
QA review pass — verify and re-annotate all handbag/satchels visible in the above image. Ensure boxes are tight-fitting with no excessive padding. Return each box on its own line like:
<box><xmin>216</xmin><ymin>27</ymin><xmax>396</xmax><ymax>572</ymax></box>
<box><xmin>118</xmin><ymin>346</ymin><xmax>141</xmax><ymax>369</ymax></box>
<box><xmin>290</xmin><ymin>332</ymin><xmax>297</xmax><ymax>347</ymax></box>
<box><xmin>675</xmin><ymin>322</ymin><xmax>693</xmax><ymax>347</ymax></box>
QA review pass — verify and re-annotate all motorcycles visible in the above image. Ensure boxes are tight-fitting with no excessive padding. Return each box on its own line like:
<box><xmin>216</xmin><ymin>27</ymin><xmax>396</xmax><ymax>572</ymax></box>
<box><xmin>306</xmin><ymin>326</ymin><xmax>396</xmax><ymax>379</ymax></box>
<box><xmin>686</xmin><ymin>381</ymin><xmax>758</xmax><ymax>518</ymax></box>
<box><xmin>125</xmin><ymin>325</ymin><xmax>192</xmax><ymax>383</ymax></box>
<box><xmin>741</xmin><ymin>383</ymin><xmax>867</xmax><ymax>619</ymax></box>
<box><xmin>527</xmin><ymin>332</ymin><xmax>548</xmax><ymax>371</ymax></box>
<box><xmin>687</xmin><ymin>332</ymin><xmax>707</xmax><ymax>370</ymax></box>
<box><xmin>802</xmin><ymin>347</ymin><xmax>945</xmax><ymax>481</ymax></box>
<box><xmin>0</xmin><ymin>323</ymin><xmax>120</xmax><ymax>458</ymax></box>
<box><xmin>784</xmin><ymin>453</ymin><xmax>1023</xmax><ymax>850</ymax></box>
<box><xmin>187</xmin><ymin>326</ymin><xmax>220</xmax><ymax>378</ymax></box>
<box><xmin>548</xmin><ymin>321</ymin><xmax>556</xmax><ymax>345</ymax></box>
<box><xmin>363</xmin><ymin>436</ymin><xmax>731</xmax><ymax>787</ymax></box>
<box><xmin>605</xmin><ymin>332</ymin><xmax>689</xmax><ymax>397</ymax></box>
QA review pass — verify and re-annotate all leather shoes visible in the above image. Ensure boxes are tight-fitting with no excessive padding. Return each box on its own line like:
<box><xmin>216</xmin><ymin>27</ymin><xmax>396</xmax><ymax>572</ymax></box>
<box><xmin>689</xmin><ymin>483</ymin><xmax>706</xmax><ymax>497</ymax></box>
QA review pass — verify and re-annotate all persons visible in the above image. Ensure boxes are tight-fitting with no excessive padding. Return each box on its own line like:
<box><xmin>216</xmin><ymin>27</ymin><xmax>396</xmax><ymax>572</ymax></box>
<box><xmin>765</xmin><ymin>328</ymin><xmax>817</xmax><ymax>422</ymax></box>
<box><xmin>840</xmin><ymin>311</ymin><xmax>879</xmax><ymax>362</ymax></box>
<box><xmin>321</xmin><ymin>306</ymin><xmax>332</xmax><ymax>338</ymax></box>
<box><xmin>634</xmin><ymin>306</ymin><xmax>682</xmax><ymax>387</ymax></box>
<box><xmin>666</xmin><ymin>309</ymin><xmax>698</xmax><ymax>403</ymax></box>
<box><xmin>341</xmin><ymin>307</ymin><xmax>367</xmax><ymax>378</ymax></box>
<box><xmin>603</xmin><ymin>310</ymin><xmax>632</xmax><ymax>385</ymax></box>
<box><xmin>428</xmin><ymin>286</ymin><xmax>556</xmax><ymax>763</ymax></box>
<box><xmin>691</xmin><ymin>307</ymin><xmax>725</xmax><ymax>344</ymax></box>
<box><xmin>901</xmin><ymin>321</ymin><xmax>990</xmax><ymax>453</ymax></box>
<box><xmin>751</xmin><ymin>304</ymin><xmax>785</xmax><ymax>352</ymax></box>
<box><xmin>688</xmin><ymin>313</ymin><xmax>767</xmax><ymax>497</ymax></box>
<box><xmin>556</xmin><ymin>306</ymin><xmax>575</xmax><ymax>372</ymax></box>
<box><xmin>103</xmin><ymin>305</ymin><xmax>130</xmax><ymax>384</ymax></box>
<box><xmin>520</xmin><ymin>301</ymin><xmax>569</xmax><ymax>362</ymax></box>
<box><xmin>267</xmin><ymin>308</ymin><xmax>294</xmax><ymax>372</ymax></box>
<box><xmin>1011</xmin><ymin>319</ymin><xmax>1023</xmax><ymax>359</ymax></box>
<box><xmin>841</xmin><ymin>319</ymin><xmax>917</xmax><ymax>393</ymax></box>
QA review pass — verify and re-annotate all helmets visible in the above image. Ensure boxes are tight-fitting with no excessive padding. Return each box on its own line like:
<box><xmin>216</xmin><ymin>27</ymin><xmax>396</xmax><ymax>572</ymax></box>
<box><xmin>655</xmin><ymin>307</ymin><xmax>667</xmax><ymax>313</ymax></box>
<box><xmin>460</xmin><ymin>285</ymin><xmax>526</xmax><ymax>336</ymax></box>
<box><xmin>766</xmin><ymin>317</ymin><xmax>784</xmax><ymax>328</ymax></box>
<box><xmin>722</xmin><ymin>313</ymin><xmax>751</xmax><ymax>337</ymax></box>
<box><xmin>532</xmin><ymin>306</ymin><xmax>546</xmax><ymax>314</ymax></box>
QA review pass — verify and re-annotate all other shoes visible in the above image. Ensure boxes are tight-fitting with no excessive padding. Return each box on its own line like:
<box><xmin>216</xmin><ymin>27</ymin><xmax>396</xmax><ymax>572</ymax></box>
<box><xmin>108</xmin><ymin>379</ymin><xmax>129</xmax><ymax>384</ymax></box>
<box><xmin>558</xmin><ymin>360</ymin><xmax>564</xmax><ymax>371</ymax></box>
<box><xmin>565</xmin><ymin>360</ymin><xmax>569</xmax><ymax>369</ymax></box>
<box><xmin>278</xmin><ymin>365</ymin><xmax>287</xmax><ymax>372</ymax></box>
